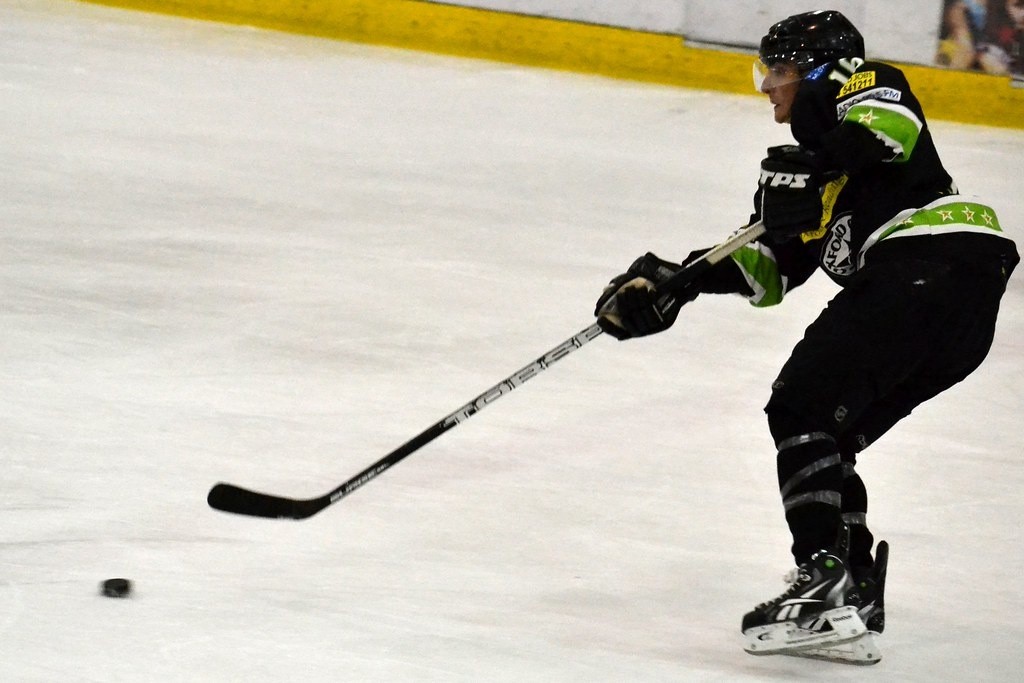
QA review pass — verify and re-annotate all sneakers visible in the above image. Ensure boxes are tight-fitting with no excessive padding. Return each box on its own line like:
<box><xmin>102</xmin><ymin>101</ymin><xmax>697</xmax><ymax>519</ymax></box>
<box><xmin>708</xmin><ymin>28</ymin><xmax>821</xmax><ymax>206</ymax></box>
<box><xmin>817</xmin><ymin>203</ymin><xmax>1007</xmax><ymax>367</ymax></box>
<box><xmin>775</xmin><ymin>540</ymin><xmax>889</xmax><ymax>667</ymax></box>
<box><xmin>739</xmin><ymin>541</ymin><xmax>867</xmax><ymax>652</ymax></box>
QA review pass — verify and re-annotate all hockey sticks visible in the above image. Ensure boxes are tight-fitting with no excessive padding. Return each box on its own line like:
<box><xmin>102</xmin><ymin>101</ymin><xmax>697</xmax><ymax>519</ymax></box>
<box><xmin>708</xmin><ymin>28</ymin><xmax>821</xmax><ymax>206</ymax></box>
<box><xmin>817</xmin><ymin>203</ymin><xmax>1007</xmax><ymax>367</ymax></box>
<box><xmin>207</xmin><ymin>218</ymin><xmax>766</xmax><ymax>522</ymax></box>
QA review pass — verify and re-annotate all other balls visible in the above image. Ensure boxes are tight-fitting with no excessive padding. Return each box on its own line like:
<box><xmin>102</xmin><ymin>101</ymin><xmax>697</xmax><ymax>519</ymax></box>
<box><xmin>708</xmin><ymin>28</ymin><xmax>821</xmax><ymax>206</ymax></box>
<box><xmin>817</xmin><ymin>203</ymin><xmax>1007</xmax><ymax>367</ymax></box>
<box><xmin>102</xmin><ymin>579</ymin><xmax>130</xmax><ymax>598</ymax></box>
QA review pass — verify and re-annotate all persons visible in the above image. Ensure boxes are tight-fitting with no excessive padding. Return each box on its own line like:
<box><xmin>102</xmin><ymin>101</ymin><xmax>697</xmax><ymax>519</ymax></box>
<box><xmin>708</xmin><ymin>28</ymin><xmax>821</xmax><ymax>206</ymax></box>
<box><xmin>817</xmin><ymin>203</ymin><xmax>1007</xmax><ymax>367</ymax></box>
<box><xmin>593</xmin><ymin>7</ymin><xmax>1021</xmax><ymax>667</ymax></box>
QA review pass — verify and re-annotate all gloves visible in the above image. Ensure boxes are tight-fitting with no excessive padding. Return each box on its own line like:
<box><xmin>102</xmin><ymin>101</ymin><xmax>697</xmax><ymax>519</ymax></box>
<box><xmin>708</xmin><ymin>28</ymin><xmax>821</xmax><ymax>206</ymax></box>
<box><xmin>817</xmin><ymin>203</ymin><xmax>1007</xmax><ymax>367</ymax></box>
<box><xmin>754</xmin><ymin>141</ymin><xmax>823</xmax><ymax>237</ymax></box>
<box><xmin>595</xmin><ymin>251</ymin><xmax>701</xmax><ymax>341</ymax></box>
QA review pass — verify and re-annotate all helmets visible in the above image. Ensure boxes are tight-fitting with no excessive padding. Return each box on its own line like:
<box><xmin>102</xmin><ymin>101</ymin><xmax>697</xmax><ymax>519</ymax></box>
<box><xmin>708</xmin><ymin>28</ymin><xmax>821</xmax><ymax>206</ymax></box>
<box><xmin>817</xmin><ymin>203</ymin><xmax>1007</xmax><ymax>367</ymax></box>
<box><xmin>758</xmin><ymin>9</ymin><xmax>866</xmax><ymax>80</ymax></box>
